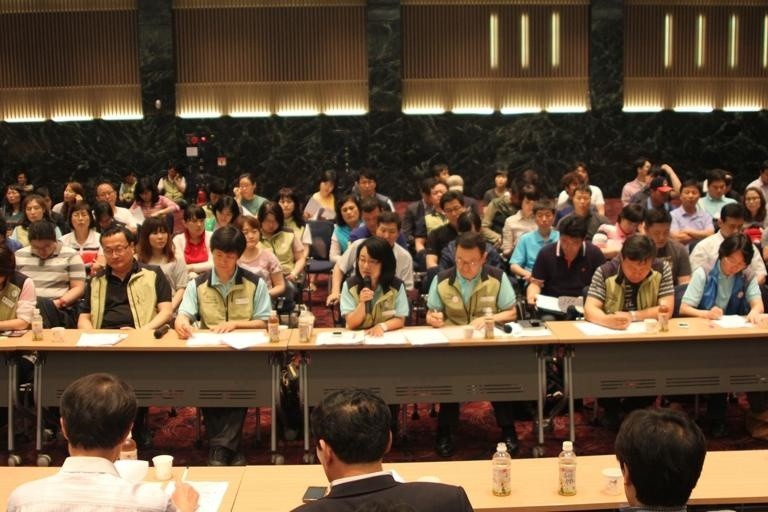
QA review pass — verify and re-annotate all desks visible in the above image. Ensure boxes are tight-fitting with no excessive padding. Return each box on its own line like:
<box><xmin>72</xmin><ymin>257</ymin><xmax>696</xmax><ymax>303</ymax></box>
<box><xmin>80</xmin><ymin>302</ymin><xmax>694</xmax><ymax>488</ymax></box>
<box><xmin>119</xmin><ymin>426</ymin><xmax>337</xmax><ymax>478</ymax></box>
<box><xmin>230</xmin><ymin>449</ymin><xmax>768</xmax><ymax>512</ymax></box>
<box><xmin>543</xmin><ymin>314</ymin><xmax>768</xmax><ymax>453</ymax></box>
<box><xmin>288</xmin><ymin>322</ymin><xmax>560</xmax><ymax>465</ymax></box>
<box><xmin>0</xmin><ymin>330</ymin><xmax>34</xmax><ymax>466</ymax></box>
<box><xmin>33</xmin><ymin>329</ymin><xmax>293</xmax><ymax>466</ymax></box>
<box><xmin>0</xmin><ymin>467</ymin><xmax>247</xmax><ymax>512</ymax></box>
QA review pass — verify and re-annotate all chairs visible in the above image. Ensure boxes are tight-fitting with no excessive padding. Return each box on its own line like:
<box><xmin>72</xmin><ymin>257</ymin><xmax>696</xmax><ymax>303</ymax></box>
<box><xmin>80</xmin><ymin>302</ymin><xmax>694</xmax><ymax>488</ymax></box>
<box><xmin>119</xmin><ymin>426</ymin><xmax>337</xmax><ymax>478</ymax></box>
<box><xmin>392</xmin><ymin>198</ymin><xmax>686</xmax><ymax>226</ymax></box>
<box><xmin>582</xmin><ymin>285</ymin><xmax>590</xmax><ymax>305</ymax></box>
<box><xmin>416</xmin><ymin>276</ymin><xmax>526</xmax><ymax>417</ymax></box>
<box><xmin>304</xmin><ymin>220</ymin><xmax>335</xmax><ymax>311</ymax></box>
<box><xmin>328</xmin><ymin>296</ymin><xmax>420</xmax><ymax>444</ymax></box>
<box><xmin>36</xmin><ymin>296</ymin><xmax>62</xmax><ymax>329</ymax></box>
<box><xmin>689</xmin><ymin>240</ymin><xmax>701</xmax><ymax>255</ymax></box>
<box><xmin>675</xmin><ymin>283</ymin><xmax>690</xmax><ymax>317</ymax></box>
<box><xmin>694</xmin><ymin>393</ymin><xmax>703</xmax><ymax>423</ymax></box>
<box><xmin>197</xmin><ymin>407</ymin><xmax>261</xmax><ymax>449</ymax></box>
<box><xmin>514</xmin><ymin>274</ymin><xmax>521</xmax><ymax>288</ymax></box>
<box><xmin>282</xmin><ymin>227</ymin><xmax>294</xmax><ymax>233</ymax></box>
<box><xmin>274</xmin><ymin>280</ymin><xmax>300</xmax><ymax>314</ymax></box>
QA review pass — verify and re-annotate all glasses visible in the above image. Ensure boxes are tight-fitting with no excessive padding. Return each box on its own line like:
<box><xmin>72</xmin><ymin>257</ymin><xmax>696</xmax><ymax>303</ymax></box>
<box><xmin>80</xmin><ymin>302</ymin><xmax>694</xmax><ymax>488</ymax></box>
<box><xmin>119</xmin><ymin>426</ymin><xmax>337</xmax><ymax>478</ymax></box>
<box><xmin>454</xmin><ymin>259</ymin><xmax>483</xmax><ymax>267</ymax></box>
<box><xmin>357</xmin><ymin>256</ymin><xmax>382</xmax><ymax>266</ymax></box>
<box><xmin>102</xmin><ymin>243</ymin><xmax>131</xmax><ymax>254</ymax></box>
<box><xmin>723</xmin><ymin>255</ymin><xmax>746</xmax><ymax>268</ymax></box>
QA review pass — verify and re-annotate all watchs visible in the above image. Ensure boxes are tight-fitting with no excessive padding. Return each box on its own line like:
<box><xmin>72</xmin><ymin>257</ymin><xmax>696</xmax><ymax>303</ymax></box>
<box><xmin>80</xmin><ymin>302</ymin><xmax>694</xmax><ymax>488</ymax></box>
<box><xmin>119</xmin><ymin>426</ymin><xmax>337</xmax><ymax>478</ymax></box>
<box><xmin>628</xmin><ymin>309</ymin><xmax>638</xmax><ymax>323</ymax></box>
<box><xmin>59</xmin><ymin>297</ymin><xmax>67</xmax><ymax>309</ymax></box>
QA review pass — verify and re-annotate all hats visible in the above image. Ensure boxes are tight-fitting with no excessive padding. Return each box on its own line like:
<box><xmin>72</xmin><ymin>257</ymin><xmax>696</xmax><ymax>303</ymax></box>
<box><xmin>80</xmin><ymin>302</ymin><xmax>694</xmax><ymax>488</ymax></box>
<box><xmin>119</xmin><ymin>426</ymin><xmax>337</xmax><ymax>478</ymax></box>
<box><xmin>650</xmin><ymin>177</ymin><xmax>675</xmax><ymax>193</ymax></box>
<box><xmin>446</xmin><ymin>174</ymin><xmax>464</xmax><ymax>192</ymax></box>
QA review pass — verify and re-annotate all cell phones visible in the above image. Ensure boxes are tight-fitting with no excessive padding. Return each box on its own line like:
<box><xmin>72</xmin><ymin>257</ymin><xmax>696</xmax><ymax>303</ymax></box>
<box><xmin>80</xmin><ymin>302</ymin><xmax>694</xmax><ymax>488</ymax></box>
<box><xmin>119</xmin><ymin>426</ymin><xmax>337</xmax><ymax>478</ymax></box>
<box><xmin>303</xmin><ymin>486</ymin><xmax>326</xmax><ymax>503</ymax></box>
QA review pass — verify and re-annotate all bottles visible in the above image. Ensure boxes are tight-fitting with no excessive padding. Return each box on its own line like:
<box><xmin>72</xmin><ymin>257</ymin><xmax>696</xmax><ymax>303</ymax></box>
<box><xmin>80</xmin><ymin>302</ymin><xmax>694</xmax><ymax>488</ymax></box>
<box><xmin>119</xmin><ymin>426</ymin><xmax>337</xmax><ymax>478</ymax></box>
<box><xmin>658</xmin><ymin>301</ymin><xmax>670</xmax><ymax>333</ymax></box>
<box><xmin>118</xmin><ymin>430</ymin><xmax>137</xmax><ymax>461</ymax></box>
<box><xmin>32</xmin><ymin>309</ymin><xmax>43</xmax><ymax>341</ymax></box>
<box><xmin>298</xmin><ymin>310</ymin><xmax>310</xmax><ymax>343</ymax></box>
<box><xmin>268</xmin><ymin>310</ymin><xmax>279</xmax><ymax>342</ymax></box>
<box><xmin>493</xmin><ymin>443</ymin><xmax>511</xmax><ymax>496</ymax></box>
<box><xmin>485</xmin><ymin>307</ymin><xmax>493</xmax><ymax>340</ymax></box>
<box><xmin>558</xmin><ymin>441</ymin><xmax>577</xmax><ymax>496</ymax></box>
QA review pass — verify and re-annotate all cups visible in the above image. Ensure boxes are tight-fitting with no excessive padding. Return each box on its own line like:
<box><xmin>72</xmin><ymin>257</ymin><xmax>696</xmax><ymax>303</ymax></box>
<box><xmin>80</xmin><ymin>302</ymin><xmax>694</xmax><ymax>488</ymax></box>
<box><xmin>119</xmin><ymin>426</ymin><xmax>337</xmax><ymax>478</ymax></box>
<box><xmin>642</xmin><ymin>317</ymin><xmax>659</xmax><ymax>335</ymax></box>
<box><xmin>151</xmin><ymin>452</ymin><xmax>176</xmax><ymax>481</ymax></box>
<box><xmin>462</xmin><ymin>327</ymin><xmax>475</xmax><ymax>339</ymax></box>
<box><xmin>599</xmin><ymin>466</ymin><xmax>625</xmax><ymax>498</ymax></box>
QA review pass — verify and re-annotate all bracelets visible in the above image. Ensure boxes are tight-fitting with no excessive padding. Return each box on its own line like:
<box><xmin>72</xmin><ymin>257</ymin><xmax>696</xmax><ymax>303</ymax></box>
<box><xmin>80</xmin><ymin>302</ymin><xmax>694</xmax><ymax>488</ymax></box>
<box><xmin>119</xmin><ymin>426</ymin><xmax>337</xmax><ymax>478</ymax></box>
<box><xmin>379</xmin><ymin>321</ymin><xmax>389</xmax><ymax>334</ymax></box>
<box><xmin>290</xmin><ymin>272</ymin><xmax>301</xmax><ymax>279</ymax></box>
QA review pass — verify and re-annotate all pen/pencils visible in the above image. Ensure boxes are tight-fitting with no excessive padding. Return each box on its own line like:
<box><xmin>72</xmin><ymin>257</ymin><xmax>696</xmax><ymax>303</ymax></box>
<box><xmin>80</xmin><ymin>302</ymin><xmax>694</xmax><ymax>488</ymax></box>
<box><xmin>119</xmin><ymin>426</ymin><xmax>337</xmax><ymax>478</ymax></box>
<box><xmin>180</xmin><ymin>466</ymin><xmax>189</xmax><ymax>483</ymax></box>
<box><xmin>433</xmin><ymin>307</ymin><xmax>442</xmax><ymax>323</ymax></box>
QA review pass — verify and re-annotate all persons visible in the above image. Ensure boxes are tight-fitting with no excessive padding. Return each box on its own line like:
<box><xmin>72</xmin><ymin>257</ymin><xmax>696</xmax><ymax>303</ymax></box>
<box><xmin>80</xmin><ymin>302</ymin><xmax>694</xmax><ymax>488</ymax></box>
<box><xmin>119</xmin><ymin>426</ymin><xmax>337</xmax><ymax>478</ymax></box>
<box><xmin>15</xmin><ymin>174</ymin><xmax>33</xmax><ymax>192</ymax></box>
<box><xmin>616</xmin><ymin>409</ymin><xmax>706</xmax><ymax>512</ymax></box>
<box><xmin>203</xmin><ymin>196</ymin><xmax>237</xmax><ymax>231</ymax></box>
<box><xmin>157</xmin><ymin>162</ymin><xmax>189</xmax><ymax>210</ymax></box>
<box><xmin>351</xmin><ymin>171</ymin><xmax>395</xmax><ymax>214</ymax></box>
<box><xmin>499</xmin><ymin>184</ymin><xmax>539</xmax><ymax>258</ymax></box>
<box><xmin>291</xmin><ymin>387</ymin><xmax>474</xmax><ymax>512</ymax></box>
<box><xmin>428</xmin><ymin>233</ymin><xmax>519</xmax><ymax>458</ymax></box>
<box><xmin>592</xmin><ymin>204</ymin><xmax>647</xmax><ymax>261</ymax></box>
<box><xmin>231</xmin><ymin>175</ymin><xmax>267</xmax><ymax>219</ymax></box>
<box><xmin>324</xmin><ymin>213</ymin><xmax>414</xmax><ymax>305</ymax></box>
<box><xmin>559</xmin><ymin>170</ymin><xmax>584</xmax><ymax>208</ymax></box>
<box><xmin>3</xmin><ymin>184</ymin><xmax>28</xmax><ymax>224</ymax></box>
<box><xmin>629</xmin><ymin>163</ymin><xmax>683</xmax><ymax>207</ymax></box>
<box><xmin>94</xmin><ymin>201</ymin><xmax>135</xmax><ymax>235</ymax></box>
<box><xmin>51</xmin><ymin>182</ymin><xmax>85</xmax><ymax>213</ymax></box>
<box><xmin>90</xmin><ymin>178</ymin><xmax>135</xmax><ymax>227</ymax></box>
<box><xmin>521</xmin><ymin>170</ymin><xmax>542</xmax><ymax>186</ymax></box>
<box><xmin>433</xmin><ymin>162</ymin><xmax>450</xmax><ymax>180</ymax></box>
<box><xmin>130</xmin><ymin>176</ymin><xmax>180</xmax><ymax>219</ymax></box>
<box><xmin>12</xmin><ymin>221</ymin><xmax>87</xmax><ymax>328</ymax></box>
<box><xmin>7</xmin><ymin>373</ymin><xmax>199</xmax><ymax>512</ymax></box>
<box><xmin>350</xmin><ymin>200</ymin><xmax>410</xmax><ymax>251</ymax></box>
<box><xmin>276</xmin><ymin>189</ymin><xmax>311</xmax><ymax>259</ymax></box>
<box><xmin>508</xmin><ymin>200</ymin><xmax>561</xmax><ymax>292</ymax></box>
<box><xmin>1</xmin><ymin>244</ymin><xmax>42</xmax><ymax>383</ymax></box>
<box><xmin>445</xmin><ymin>175</ymin><xmax>464</xmax><ymax>194</ymax></box>
<box><xmin>560</xmin><ymin>187</ymin><xmax>611</xmax><ymax>240</ymax></box>
<box><xmin>118</xmin><ymin>170</ymin><xmax>139</xmax><ymax>206</ymax></box>
<box><xmin>638</xmin><ymin>176</ymin><xmax>674</xmax><ymax>213</ymax></box>
<box><xmin>79</xmin><ymin>227</ymin><xmax>174</xmax><ymax>449</ymax></box>
<box><xmin>258</xmin><ymin>200</ymin><xmax>308</xmax><ymax>310</ymax></box>
<box><xmin>414</xmin><ymin>179</ymin><xmax>452</xmax><ymax>251</ymax></box>
<box><xmin>341</xmin><ymin>236</ymin><xmax>409</xmax><ymax>338</ymax></box>
<box><xmin>671</xmin><ymin>179</ymin><xmax>713</xmax><ymax>250</ymax></box>
<box><xmin>747</xmin><ymin>161</ymin><xmax>768</xmax><ymax>202</ymax></box>
<box><xmin>687</xmin><ymin>203</ymin><xmax>766</xmax><ymax>286</ymax></box>
<box><xmin>35</xmin><ymin>185</ymin><xmax>66</xmax><ymax>231</ymax></box>
<box><xmin>232</xmin><ymin>216</ymin><xmax>284</xmax><ymax>312</ymax></box>
<box><xmin>582</xmin><ymin>234</ymin><xmax>674</xmax><ymax>432</ymax></box>
<box><xmin>137</xmin><ymin>216</ymin><xmax>188</xmax><ymax>310</ymax></box>
<box><xmin>171</xmin><ymin>205</ymin><xmax>217</xmax><ymax>280</ymax></box>
<box><xmin>302</xmin><ymin>169</ymin><xmax>341</xmax><ymax>221</ymax></box>
<box><xmin>59</xmin><ymin>203</ymin><xmax>109</xmax><ymax>277</ymax></box>
<box><xmin>197</xmin><ymin>179</ymin><xmax>228</xmax><ymax>218</ymax></box>
<box><xmin>557</xmin><ymin>163</ymin><xmax>606</xmax><ymax>215</ymax></box>
<box><xmin>639</xmin><ymin>207</ymin><xmax>692</xmax><ymax>287</ymax></box>
<box><xmin>621</xmin><ymin>159</ymin><xmax>655</xmax><ymax>208</ymax></box>
<box><xmin>482</xmin><ymin>168</ymin><xmax>508</xmax><ymax>206</ymax></box>
<box><xmin>427</xmin><ymin>191</ymin><xmax>468</xmax><ymax>270</ymax></box>
<box><xmin>695</xmin><ymin>169</ymin><xmax>742</xmax><ymax>202</ymax></box>
<box><xmin>741</xmin><ymin>188</ymin><xmax>767</xmax><ymax>260</ymax></box>
<box><xmin>699</xmin><ymin>170</ymin><xmax>738</xmax><ymax>233</ymax></box>
<box><xmin>174</xmin><ymin>224</ymin><xmax>272</xmax><ymax>467</ymax></box>
<box><xmin>482</xmin><ymin>178</ymin><xmax>531</xmax><ymax>247</ymax></box>
<box><xmin>438</xmin><ymin>211</ymin><xmax>510</xmax><ymax>273</ymax></box>
<box><xmin>681</xmin><ymin>233</ymin><xmax>764</xmax><ymax>439</ymax></box>
<box><xmin>527</xmin><ymin>212</ymin><xmax>608</xmax><ymax>414</ymax></box>
<box><xmin>329</xmin><ymin>194</ymin><xmax>366</xmax><ymax>263</ymax></box>
<box><xmin>12</xmin><ymin>195</ymin><xmax>62</xmax><ymax>245</ymax></box>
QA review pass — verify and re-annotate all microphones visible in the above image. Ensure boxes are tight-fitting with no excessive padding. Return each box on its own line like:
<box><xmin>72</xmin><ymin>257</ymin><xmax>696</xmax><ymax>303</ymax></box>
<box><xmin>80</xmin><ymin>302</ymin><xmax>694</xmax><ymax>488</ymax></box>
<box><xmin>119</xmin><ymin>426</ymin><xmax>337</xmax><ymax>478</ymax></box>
<box><xmin>363</xmin><ymin>275</ymin><xmax>373</xmax><ymax>317</ymax></box>
<box><xmin>495</xmin><ymin>321</ymin><xmax>511</xmax><ymax>332</ymax></box>
<box><xmin>154</xmin><ymin>326</ymin><xmax>171</xmax><ymax>338</ymax></box>
